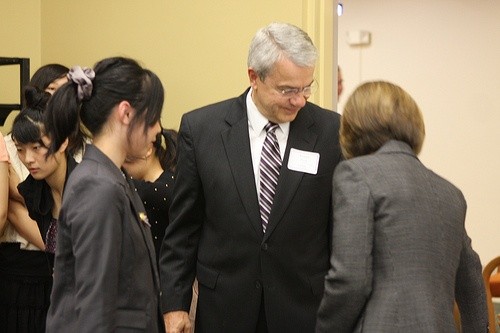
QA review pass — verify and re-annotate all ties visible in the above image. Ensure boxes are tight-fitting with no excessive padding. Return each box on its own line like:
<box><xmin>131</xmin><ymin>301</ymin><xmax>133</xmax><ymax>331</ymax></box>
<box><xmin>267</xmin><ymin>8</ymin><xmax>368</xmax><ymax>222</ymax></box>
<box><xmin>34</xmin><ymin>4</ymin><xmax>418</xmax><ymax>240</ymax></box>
<box><xmin>257</xmin><ymin>122</ymin><xmax>284</xmax><ymax>234</ymax></box>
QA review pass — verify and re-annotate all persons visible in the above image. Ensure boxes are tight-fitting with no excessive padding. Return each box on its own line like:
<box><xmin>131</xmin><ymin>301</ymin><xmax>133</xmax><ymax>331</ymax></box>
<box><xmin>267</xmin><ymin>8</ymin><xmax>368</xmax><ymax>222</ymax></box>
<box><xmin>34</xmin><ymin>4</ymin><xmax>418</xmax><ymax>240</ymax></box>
<box><xmin>315</xmin><ymin>80</ymin><xmax>489</xmax><ymax>333</ymax></box>
<box><xmin>160</xmin><ymin>22</ymin><xmax>341</xmax><ymax>332</ymax></box>
<box><xmin>0</xmin><ymin>132</ymin><xmax>10</xmax><ymax>236</ymax></box>
<box><xmin>4</xmin><ymin>63</ymin><xmax>93</xmax><ymax>251</ymax></box>
<box><xmin>122</xmin><ymin>120</ymin><xmax>178</xmax><ymax>259</ymax></box>
<box><xmin>45</xmin><ymin>57</ymin><xmax>165</xmax><ymax>333</ymax></box>
<box><xmin>11</xmin><ymin>84</ymin><xmax>81</xmax><ymax>253</ymax></box>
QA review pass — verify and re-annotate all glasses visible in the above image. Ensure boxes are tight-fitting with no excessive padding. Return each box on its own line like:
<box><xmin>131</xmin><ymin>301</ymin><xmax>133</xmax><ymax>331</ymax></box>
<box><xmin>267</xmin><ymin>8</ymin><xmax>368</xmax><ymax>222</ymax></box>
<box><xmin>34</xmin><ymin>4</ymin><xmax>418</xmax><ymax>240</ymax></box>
<box><xmin>271</xmin><ymin>86</ymin><xmax>314</xmax><ymax>97</ymax></box>
